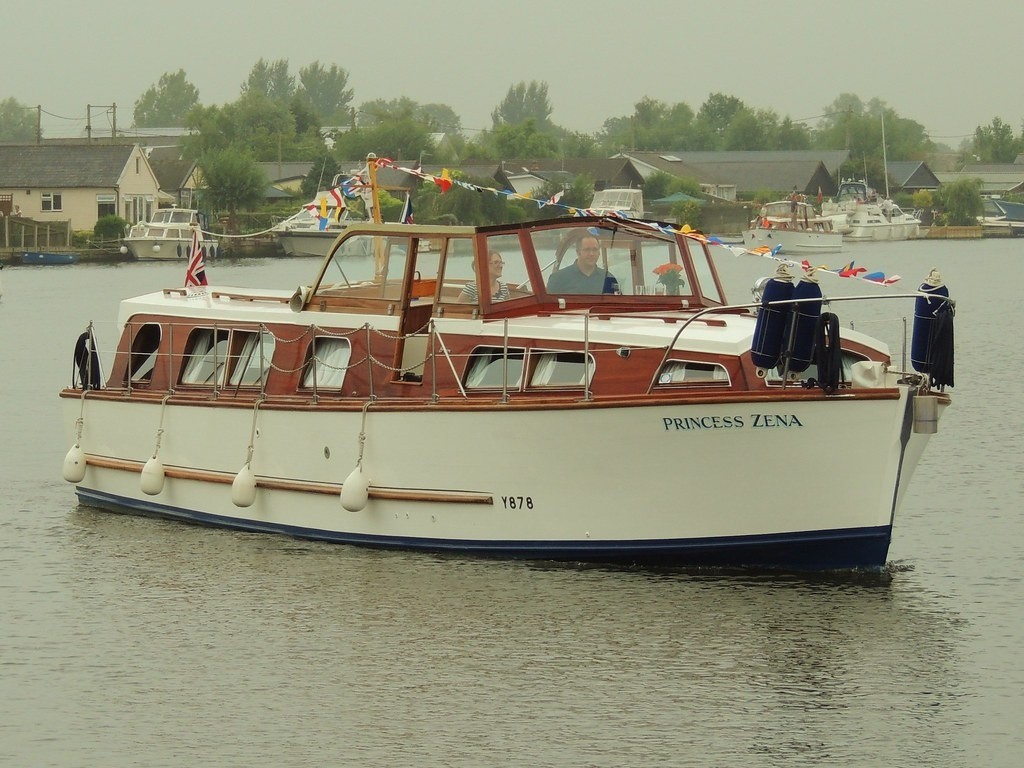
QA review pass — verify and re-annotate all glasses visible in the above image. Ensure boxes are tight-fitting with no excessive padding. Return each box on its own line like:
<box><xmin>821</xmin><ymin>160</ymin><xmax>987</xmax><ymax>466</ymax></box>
<box><xmin>489</xmin><ymin>260</ymin><xmax>506</xmax><ymax>265</ymax></box>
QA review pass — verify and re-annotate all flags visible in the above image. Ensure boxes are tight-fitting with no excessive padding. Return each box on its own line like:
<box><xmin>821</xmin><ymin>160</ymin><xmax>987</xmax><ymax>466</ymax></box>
<box><xmin>185</xmin><ymin>231</ymin><xmax>208</xmax><ymax>286</ymax></box>
<box><xmin>404</xmin><ymin>197</ymin><xmax>413</xmax><ymax>224</ymax></box>
<box><xmin>818</xmin><ymin>186</ymin><xmax>822</xmax><ymax>204</ymax></box>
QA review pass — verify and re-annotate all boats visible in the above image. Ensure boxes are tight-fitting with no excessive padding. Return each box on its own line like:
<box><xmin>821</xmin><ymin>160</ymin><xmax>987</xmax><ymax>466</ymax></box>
<box><xmin>271</xmin><ymin>169</ymin><xmax>398</xmax><ymax>255</ymax></box>
<box><xmin>121</xmin><ymin>203</ymin><xmax>218</xmax><ymax>260</ymax></box>
<box><xmin>822</xmin><ymin>114</ymin><xmax>922</xmax><ymax>242</ymax></box>
<box><xmin>574</xmin><ymin>189</ymin><xmax>644</xmax><ymax>219</ymax></box>
<box><xmin>21</xmin><ymin>251</ymin><xmax>81</xmax><ymax>264</ymax></box>
<box><xmin>742</xmin><ymin>201</ymin><xmax>843</xmax><ymax>255</ymax></box>
<box><xmin>59</xmin><ymin>153</ymin><xmax>955</xmax><ymax>573</ymax></box>
<box><xmin>992</xmin><ymin>200</ymin><xmax>1024</xmax><ymax>221</ymax></box>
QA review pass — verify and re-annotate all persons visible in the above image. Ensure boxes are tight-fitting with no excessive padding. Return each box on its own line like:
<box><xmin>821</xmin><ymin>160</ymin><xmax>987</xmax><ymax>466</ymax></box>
<box><xmin>457</xmin><ymin>250</ymin><xmax>510</xmax><ymax>304</ymax></box>
<box><xmin>14</xmin><ymin>205</ymin><xmax>22</xmax><ymax>217</ymax></box>
<box><xmin>546</xmin><ymin>233</ymin><xmax>622</xmax><ymax>293</ymax></box>
